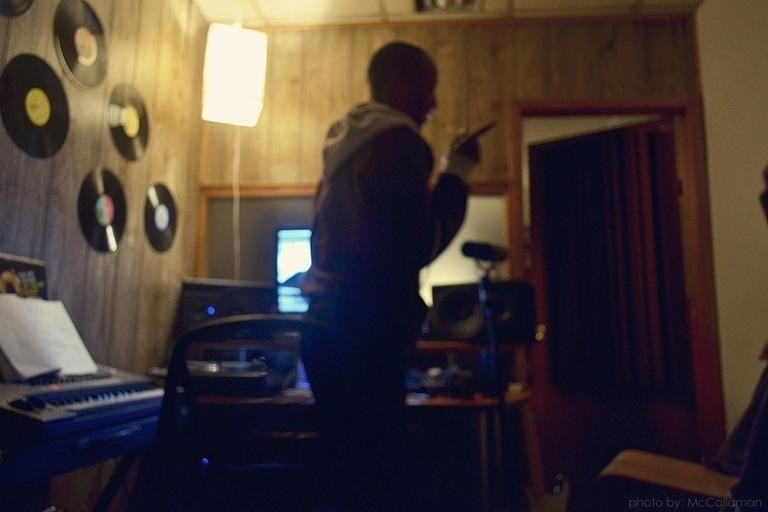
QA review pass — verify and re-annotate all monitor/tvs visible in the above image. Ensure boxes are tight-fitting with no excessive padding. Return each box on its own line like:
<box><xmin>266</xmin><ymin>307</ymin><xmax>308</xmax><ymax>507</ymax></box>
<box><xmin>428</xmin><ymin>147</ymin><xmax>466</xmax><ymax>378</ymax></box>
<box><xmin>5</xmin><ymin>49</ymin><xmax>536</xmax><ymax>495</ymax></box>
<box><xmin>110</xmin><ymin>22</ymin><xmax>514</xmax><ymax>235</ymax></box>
<box><xmin>271</xmin><ymin>225</ymin><xmax>312</xmax><ymax>314</ymax></box>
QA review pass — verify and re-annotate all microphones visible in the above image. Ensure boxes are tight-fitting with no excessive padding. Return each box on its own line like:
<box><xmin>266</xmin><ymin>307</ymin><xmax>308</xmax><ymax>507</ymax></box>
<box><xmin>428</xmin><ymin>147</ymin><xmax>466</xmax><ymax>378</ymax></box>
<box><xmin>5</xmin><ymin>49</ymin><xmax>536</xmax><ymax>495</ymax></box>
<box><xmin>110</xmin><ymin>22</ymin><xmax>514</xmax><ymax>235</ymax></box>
<box><xmin>462</xmin><ymin>242</ymin><xmax>507</xmax><ymax>262</ymax></box>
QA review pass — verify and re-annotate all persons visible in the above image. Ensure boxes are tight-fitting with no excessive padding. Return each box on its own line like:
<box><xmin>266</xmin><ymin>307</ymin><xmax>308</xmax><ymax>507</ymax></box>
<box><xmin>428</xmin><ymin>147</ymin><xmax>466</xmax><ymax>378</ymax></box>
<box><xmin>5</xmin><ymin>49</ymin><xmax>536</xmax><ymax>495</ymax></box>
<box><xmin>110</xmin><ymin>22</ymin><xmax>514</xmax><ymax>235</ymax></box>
<box><xmin>293</xmin><ymin>37</ymin><xmax>484</xmax><ymax>512</ymax></box>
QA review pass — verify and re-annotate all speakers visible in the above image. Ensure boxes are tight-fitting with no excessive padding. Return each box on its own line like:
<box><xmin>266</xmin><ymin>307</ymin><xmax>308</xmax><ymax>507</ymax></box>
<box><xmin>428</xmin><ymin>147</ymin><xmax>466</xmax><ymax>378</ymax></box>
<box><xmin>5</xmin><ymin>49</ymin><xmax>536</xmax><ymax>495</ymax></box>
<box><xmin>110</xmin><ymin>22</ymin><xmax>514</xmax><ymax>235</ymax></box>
<box><xmin>432</xmin><ymin>280</ymin><xmax>537</xmax><ymax>345</ymax></box>
<box><xmin>182</xmin><ymin>276</ymin><xmax>288</xmax><ymax>340</ymax></box>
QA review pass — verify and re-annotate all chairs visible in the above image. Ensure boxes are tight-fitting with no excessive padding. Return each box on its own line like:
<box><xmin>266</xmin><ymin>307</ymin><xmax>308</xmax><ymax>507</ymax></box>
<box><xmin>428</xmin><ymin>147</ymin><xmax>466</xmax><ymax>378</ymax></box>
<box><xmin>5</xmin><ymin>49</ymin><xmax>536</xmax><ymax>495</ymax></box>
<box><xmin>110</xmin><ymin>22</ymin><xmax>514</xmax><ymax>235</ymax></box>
<box><xmin>594</xmin><ymin>364</ymin><xmax>767</xmax><ymax>512</ymax></box>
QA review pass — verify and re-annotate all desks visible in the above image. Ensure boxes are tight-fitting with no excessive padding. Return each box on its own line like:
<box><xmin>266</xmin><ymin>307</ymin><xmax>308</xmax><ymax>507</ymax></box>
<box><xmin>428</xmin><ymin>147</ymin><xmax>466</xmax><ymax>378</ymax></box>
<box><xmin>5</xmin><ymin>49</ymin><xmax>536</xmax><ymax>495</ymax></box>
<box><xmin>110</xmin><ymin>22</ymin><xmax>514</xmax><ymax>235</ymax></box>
<box><xmin>161</xmin><ymin>333</ymin><xmax>546</xmax><ymax>511</ymax></box>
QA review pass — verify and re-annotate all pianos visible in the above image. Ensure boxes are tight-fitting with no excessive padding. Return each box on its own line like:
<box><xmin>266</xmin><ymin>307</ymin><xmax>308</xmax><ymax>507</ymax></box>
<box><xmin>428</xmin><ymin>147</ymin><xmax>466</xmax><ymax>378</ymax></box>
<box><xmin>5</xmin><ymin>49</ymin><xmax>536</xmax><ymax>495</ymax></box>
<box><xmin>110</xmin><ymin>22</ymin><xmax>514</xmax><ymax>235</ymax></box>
<box><xmin>0</xmin><ymin>365</ymin><xmax>164</xmax><ymax>443</ymax></box>
